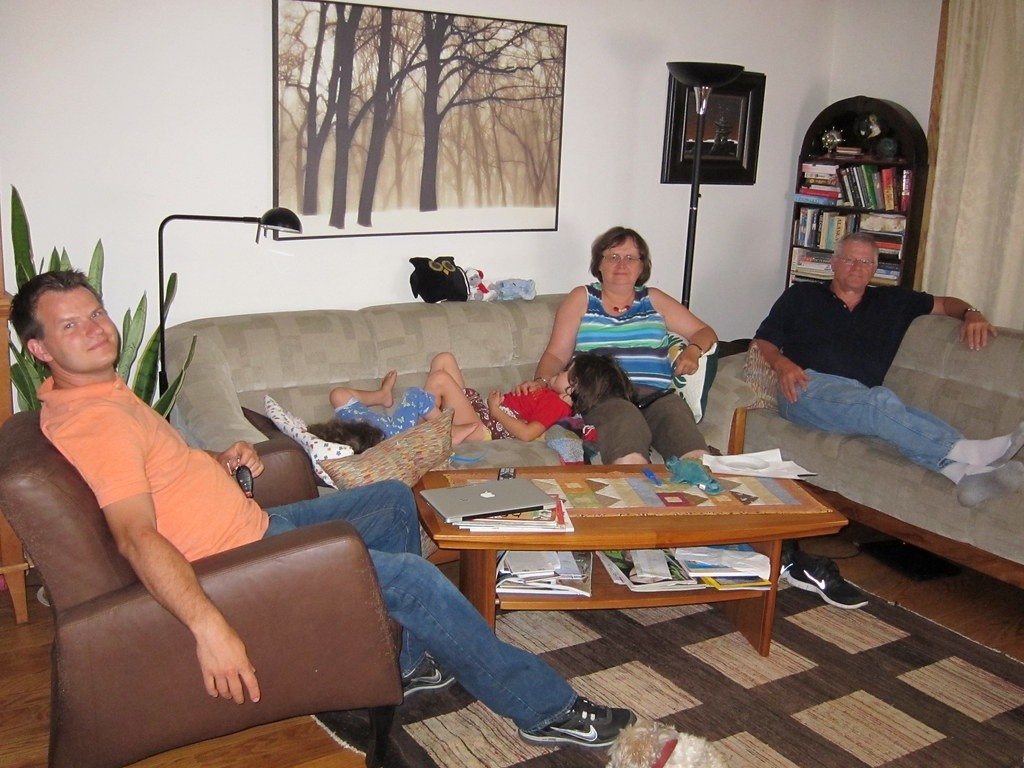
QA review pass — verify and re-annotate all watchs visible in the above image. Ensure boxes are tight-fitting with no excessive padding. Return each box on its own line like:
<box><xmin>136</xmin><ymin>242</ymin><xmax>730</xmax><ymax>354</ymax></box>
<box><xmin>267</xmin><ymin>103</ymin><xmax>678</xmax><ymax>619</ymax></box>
<box><xmin>687</xmin><ymin>343</ymin><xmax>704</xmax><ymax>358</ymax></box>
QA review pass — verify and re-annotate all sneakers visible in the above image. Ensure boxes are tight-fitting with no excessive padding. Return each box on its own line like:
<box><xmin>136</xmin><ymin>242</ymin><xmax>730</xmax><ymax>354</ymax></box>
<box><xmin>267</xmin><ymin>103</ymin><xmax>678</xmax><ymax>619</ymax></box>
<box><xmin>518</xmin><ymin>696</ymin><xmax>640</xmax><ymax>747</ymax></box>
<box><xmin>779</xmin><ymin>540</ymin><xmax>869</xmax><ymax>609</ymax></box>
<box><xmin>401</xmin><ymin>655</ymin><xmax>457</xmax><ymax>697</ymax></box>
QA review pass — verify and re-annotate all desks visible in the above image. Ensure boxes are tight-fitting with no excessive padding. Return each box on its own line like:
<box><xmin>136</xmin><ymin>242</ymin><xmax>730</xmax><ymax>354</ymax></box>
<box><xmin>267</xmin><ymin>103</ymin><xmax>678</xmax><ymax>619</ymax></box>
<box><xmin>412</xmin><ymin>462</ymin><xmax>849</xmax><ymax>659</ymax></box>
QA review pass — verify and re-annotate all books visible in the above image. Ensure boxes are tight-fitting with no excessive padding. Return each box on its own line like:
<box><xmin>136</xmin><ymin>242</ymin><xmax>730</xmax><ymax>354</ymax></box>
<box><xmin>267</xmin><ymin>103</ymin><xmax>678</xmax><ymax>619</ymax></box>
<box><xmin>453</xmin><ymin>479</ymin><xmax>574</xmax><ymax>533</ymax></box>
<box><xmin>496</xmin><ymin>551</ymin><xmax>593</xmax><ymax>600</ymax></box>
<box><xmin>595</xmin><ymin>545</ymin><xmax>772</xmax><ymax>592</ymax></box>
<box><xmin>799</xmin><ymin>160</ymin><xmax>914</xmax><ymax>215</ymax></box>
<box><xmin>788</xmin><ymin>208</ymin><xmax>906</xmax><ymax>290</ymax></box>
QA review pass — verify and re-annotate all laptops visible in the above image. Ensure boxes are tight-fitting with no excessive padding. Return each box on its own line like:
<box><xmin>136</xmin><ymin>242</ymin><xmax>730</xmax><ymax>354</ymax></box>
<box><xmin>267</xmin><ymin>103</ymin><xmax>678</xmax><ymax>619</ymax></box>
<box><xmin>419</xmin><ymin>477</ymin><xmax>557</xmax><ymax>523</ymax></box>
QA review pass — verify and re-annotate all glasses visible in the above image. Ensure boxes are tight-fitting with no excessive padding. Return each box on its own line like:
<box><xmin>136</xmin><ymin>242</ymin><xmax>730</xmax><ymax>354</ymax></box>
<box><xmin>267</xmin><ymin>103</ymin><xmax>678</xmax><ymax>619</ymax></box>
<box><xmin>837</xmin><ymin>255</ymin><xmax>874</xmax><ymax>266</ymax></box>
<box><xmin>602</xmin><ymin>254</ymin><xmax>641</xmax><ymax>264</ymax></box>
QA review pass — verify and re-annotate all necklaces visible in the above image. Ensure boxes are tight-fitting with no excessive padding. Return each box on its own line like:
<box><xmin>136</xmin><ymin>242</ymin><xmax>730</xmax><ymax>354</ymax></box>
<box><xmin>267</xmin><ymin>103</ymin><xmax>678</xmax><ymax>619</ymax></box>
<box><xmin>608</xmin><ymin>301</ymin><xmax>631</xmax><ymax>313</ymax></box>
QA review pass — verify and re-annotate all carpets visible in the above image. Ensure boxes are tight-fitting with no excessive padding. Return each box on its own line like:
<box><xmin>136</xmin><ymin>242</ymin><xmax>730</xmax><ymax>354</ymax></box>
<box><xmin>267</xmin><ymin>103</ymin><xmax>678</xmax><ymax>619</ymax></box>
<box><xmin>301</xmin><ymin>544</ymin><xmax>1024</xmax><ymax>768</ymax></box>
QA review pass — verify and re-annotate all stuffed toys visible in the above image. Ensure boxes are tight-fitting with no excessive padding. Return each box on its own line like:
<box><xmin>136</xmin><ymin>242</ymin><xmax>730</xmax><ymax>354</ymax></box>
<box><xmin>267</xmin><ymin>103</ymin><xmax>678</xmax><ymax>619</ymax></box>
<box><xmin>409</xmin><ymin>257</ymin><xmax>537</xmax><ymax>303</ymax></box>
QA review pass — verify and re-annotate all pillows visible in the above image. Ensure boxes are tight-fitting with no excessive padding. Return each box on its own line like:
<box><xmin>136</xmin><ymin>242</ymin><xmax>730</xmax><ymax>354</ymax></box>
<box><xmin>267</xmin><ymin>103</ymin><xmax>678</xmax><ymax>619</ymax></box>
<box><xmin>665</xmin><ymin>330</ymin><xmax>720</xmax><ymax>426</ymax></box>
<box><xmin>740</xmin><ymin>336</ymin><xmax>784</xmax><ymax>411</ymax></box>
<box><xmin>317</xmin><ymin>406</ymin><xmax>454</xmax><ymax>490</ymax></box>
<box><xmin>240</xmin><ymin>394</ymin><xmax>353</xmax><ymax>490</ymax></box>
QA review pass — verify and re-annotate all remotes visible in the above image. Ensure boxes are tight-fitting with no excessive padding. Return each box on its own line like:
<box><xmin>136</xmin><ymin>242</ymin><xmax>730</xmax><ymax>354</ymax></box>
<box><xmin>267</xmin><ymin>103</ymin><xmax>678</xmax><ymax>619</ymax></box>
<box><xmin>636</xmin><ymin>388</ymin><xmax>675</xmax><ymax>408</ymax></box>
<box><xmin>498</xmin><ymin>467</ymin><xmax>516</xmax><ymax>480</ymax></box>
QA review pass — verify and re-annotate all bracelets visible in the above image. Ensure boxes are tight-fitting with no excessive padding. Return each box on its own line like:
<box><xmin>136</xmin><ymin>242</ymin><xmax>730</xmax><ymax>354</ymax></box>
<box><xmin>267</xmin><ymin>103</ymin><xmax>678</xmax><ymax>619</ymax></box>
<box><xmin>534</xmin><ymin>377</ymin><xmax>548</xmax><ymax>388</ymax></box>
<box><xmin>962</xmin><ymin>308</ymin><xmax>980</xmax><ymax>320</ymax></box>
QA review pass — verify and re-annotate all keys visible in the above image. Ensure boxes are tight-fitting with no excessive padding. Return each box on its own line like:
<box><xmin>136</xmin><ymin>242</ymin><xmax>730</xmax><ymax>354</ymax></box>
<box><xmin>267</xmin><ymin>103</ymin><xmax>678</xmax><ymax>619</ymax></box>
<box><xmin>226</xmin><ymin>459</ymin><xmax>253</xmax><ymax>498</ymax></box>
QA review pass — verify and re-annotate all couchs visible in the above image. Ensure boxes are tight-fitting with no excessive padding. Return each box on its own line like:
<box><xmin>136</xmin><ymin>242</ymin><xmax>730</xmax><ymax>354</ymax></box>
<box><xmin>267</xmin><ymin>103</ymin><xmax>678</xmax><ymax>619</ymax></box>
<box><xmin>161</xmin><ymin>294</ymin><xmax>727</xmax><ymax>568</ymax></box>
<box><xmin>696</xmin><ymin>312</ymin><xmax>1024</xmax><ymax>597</ymax></box>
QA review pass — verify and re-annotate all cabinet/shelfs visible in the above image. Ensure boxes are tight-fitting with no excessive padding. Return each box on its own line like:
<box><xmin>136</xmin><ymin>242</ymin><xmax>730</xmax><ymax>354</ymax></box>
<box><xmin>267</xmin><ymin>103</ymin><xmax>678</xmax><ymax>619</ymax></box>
<box><xmin>785</xmin><ymin>97</ymin><xmax>930</xmax><ymax>295</ymax></box>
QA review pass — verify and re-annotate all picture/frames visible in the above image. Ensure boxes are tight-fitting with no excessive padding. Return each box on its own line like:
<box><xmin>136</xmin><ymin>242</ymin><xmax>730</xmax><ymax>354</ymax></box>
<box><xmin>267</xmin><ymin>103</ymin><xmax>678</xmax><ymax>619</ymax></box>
<box><xmin>271</xmin><ymin>0</ymin><xmax>568</xmax><ymax>243</ymax></box>
<box><xmin>657</xmin><ymin>68</ymin><xmax>768</xmax><ymax>187</ymax></box>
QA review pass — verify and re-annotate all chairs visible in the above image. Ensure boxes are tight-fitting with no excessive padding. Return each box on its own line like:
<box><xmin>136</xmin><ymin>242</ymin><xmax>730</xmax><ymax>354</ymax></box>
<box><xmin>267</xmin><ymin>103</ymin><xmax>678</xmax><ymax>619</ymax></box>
<box><xmin>0</xmin><ymin>408</ymin><xmax>405</xmax><ymax>768</ymax></box>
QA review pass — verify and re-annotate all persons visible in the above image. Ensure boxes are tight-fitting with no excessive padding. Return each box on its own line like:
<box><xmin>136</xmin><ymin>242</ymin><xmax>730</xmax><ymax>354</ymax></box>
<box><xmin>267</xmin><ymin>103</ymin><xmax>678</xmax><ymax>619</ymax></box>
<box><xmin>511</xmin><ymin>226</ymin><xmax>719</xmax><ymax>464</ymax></box>
<box><xmin>422</xmin><ymin>351</ymin><xmax>634</xmax><ymax>442</ymax></box>
<box><xmin>307</xmin><ymin>370</ymin><xmax>479</xmax><ymax>455</ymax></box>
<box><xmin>8</xmin><ymin>267</ymin><xmax>638</xmax><ymax>751</ymax></box>
<box><xmin>749</xmin><ymin>233</ymin><xmax>1024</xmax><ymax>507</ymax></box>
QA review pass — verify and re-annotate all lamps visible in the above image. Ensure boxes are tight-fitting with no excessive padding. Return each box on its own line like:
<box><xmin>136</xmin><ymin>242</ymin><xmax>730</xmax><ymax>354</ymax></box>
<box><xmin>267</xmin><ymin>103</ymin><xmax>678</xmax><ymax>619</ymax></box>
<box><xmin>156</xmin><ymin>207</ymin><xmax>302</xmax><ymax>425</ymax></box>
<box><xmin>666</xmin><ymin>61</ymin><xmax>744</xmax><ymax>311</ymax></box>
